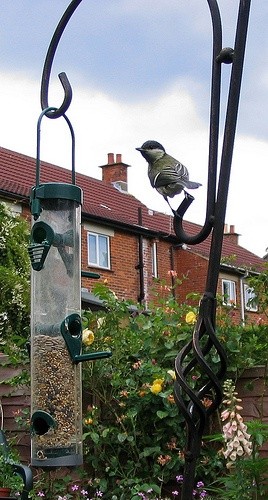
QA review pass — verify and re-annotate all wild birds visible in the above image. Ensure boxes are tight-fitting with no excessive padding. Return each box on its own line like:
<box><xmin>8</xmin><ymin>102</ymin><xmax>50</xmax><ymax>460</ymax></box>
<box><xmin>136</xmin><ymin>141</ymin><xmax>203</xmax><ymax>218</ymax></box>
